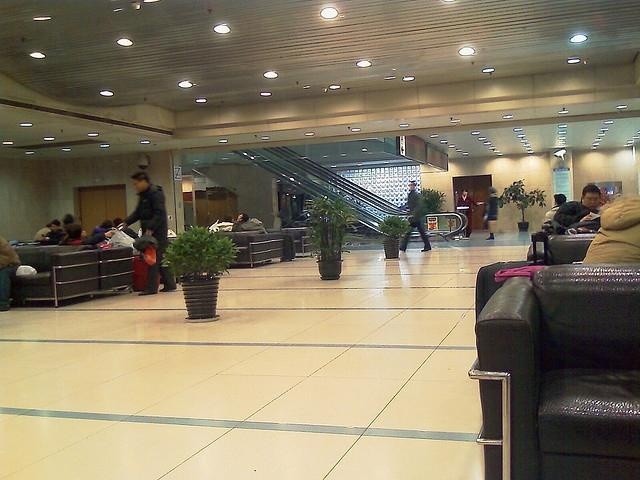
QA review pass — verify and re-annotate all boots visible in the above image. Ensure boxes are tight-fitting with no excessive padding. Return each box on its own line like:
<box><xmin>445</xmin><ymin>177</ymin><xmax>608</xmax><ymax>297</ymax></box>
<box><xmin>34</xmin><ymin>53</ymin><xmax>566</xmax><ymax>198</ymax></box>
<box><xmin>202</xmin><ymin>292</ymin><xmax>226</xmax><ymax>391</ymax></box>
<box><xmin>485</xmin><ymin>233</ymin><xmax>494</xmax><ymax>240</ymax></box>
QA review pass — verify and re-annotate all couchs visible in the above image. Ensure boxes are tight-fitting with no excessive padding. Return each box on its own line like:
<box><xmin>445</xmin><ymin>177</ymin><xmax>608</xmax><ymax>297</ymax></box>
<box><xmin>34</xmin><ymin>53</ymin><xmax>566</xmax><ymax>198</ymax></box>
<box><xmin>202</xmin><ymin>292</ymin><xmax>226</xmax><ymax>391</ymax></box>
<box><xmin>466</xmin><ymin>263</ymin><xmax>640</xmax><ymax>480</ymax></box>
<box><xmin>218</xmin><ymin>230</ymin><xmax>289</xmax><ymax>268</ymax></box>
<box><xmin>12</xmin><ymin>250</ymin><xmax>100</xmax><ymax>308</ymax></box>
<box><xmin>12</xmin><ymin>244</ymin><xmax>93</xmax><ymax>271</ymax></box>
<box><xmin>98</xmin><ymin>246</ymin><xmax>136</xmax><ymax>294</ymax></box>
<box><xmin>283</xmin><ymin>226</ymin><xmax>315</xmax><ymax>258</ymax></box>
<box><xmin>546</xmin><ymin>235</ymin><xmax>596</xmax><ymax>266</ymax></box>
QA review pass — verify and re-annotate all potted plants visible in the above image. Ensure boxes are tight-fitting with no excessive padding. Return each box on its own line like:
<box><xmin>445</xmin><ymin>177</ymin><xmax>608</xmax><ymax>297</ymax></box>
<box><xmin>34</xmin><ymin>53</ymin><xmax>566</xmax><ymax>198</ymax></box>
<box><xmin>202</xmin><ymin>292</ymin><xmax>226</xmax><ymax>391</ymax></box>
<box><xmin>307</xmin><ymin>196</ymin><xmax>354</xmax><ymax>281</ymax></box>
<box><xmin>379</xmin><ymin>216</ymin><xmax>411</xmax><ymax>261</ymax></box>
<box><xmin>160</xmin><ymin>224</ymin><xmax>239</xmax><ymax>324</ymax></box>
<box><xmin>498</xmin><ymin>179</ymin><xmax>547</xmax><ymax>233</ymax></box>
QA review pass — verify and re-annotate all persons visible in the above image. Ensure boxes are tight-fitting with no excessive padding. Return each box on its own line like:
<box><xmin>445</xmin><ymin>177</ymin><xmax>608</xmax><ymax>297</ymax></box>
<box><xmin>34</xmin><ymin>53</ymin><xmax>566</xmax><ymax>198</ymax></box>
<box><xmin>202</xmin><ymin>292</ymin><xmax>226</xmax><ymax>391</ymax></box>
<box><xmin>399</xmin><ymin>181</ymin><xmax>431</xmax><ymax>252</ymax></box>
<box><xmin>457</xmin><ymin>188</ymin><xmax>472</xmax><ymax>238</ymax></box>
<box><xmin>545</xmin><ymin>194</ymin><xmax>566</xmax><ymax>221</ymax></box>
<box><xmin>584</xmin><ymin>194</ymin><xmax>640</xmax><ymax>265</ymax></box>
<box><xmin>34</xmin><ymin>213</ymin><xmax>122</xmax><ymax>248</ymax></box>
<box><xmin>233</xmin><ymin>212</ymin><xmax>248</xmax><ymax>232</ymax></box>
<box><xmin>553</xmin><ymin>184</ymin><xmax>601</xmax><ymax>235</ymax></box>
<box><xmin>484</xmin><ymin>187</ymin><xmax>499</xmax><ymax>241</ymax></box>
<box><xmin>117</xmin><ymin>172</ymin><xmax>178</xmax><ymax>295</ymax></box>
<box><xmin>0</xmin><ymin>236</ymin><xmax>21</xmax><ymax>311</ymax></box>
